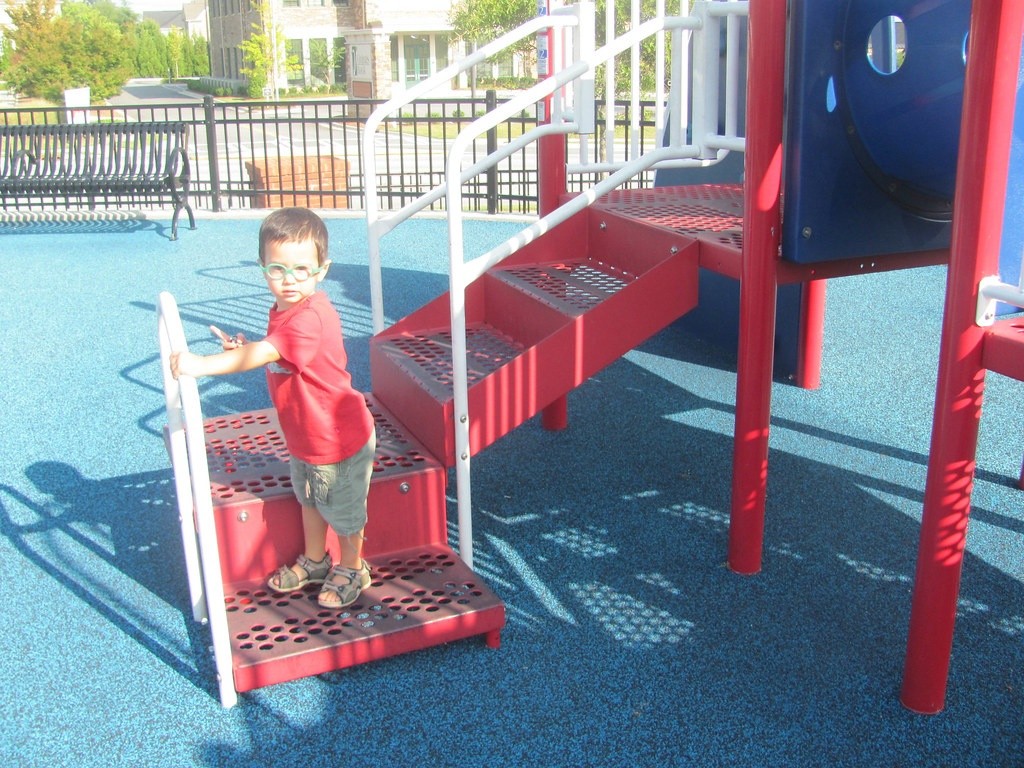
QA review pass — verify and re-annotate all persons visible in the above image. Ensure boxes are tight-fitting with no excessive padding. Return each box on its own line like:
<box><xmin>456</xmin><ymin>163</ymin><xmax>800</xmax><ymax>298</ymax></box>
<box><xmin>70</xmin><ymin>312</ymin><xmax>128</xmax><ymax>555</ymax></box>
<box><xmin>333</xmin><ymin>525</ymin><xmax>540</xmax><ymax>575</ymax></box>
<box><xmin>166</xmin><ymin>206</ymin><xmax>377</xmax><ymax>610</ymax></box>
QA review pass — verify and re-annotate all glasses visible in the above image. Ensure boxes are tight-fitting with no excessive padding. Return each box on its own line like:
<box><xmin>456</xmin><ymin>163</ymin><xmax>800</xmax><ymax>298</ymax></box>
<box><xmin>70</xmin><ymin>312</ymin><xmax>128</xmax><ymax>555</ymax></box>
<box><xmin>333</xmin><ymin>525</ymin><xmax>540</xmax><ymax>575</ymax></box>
<box><xmin>259</xmin><ymin>263</ymin><xmax>323</xmax><ymax>280</ymax></box>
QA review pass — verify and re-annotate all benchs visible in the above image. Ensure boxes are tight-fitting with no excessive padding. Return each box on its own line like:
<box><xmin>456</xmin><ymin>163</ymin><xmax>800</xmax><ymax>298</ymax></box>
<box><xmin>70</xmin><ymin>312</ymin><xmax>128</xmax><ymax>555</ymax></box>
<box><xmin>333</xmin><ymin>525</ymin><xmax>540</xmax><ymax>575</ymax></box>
<box><xmin>0</xmin><ymin>122</ymin><xmax>198</xmax><ymax>243</ymax></box>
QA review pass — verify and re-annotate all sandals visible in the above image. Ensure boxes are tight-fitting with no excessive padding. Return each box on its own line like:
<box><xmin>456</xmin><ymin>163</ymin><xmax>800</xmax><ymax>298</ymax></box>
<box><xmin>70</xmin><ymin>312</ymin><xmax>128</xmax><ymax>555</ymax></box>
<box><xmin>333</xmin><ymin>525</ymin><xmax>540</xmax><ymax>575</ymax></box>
<box><xmin>267</xmin><ymin>554</ymin><xmax>332</xmax><ymax>592</ymax></box>
<box><xmin>318</xmin><ymin>558</ymin><xmax>372</xmax><ymax>607</ymax></box>
